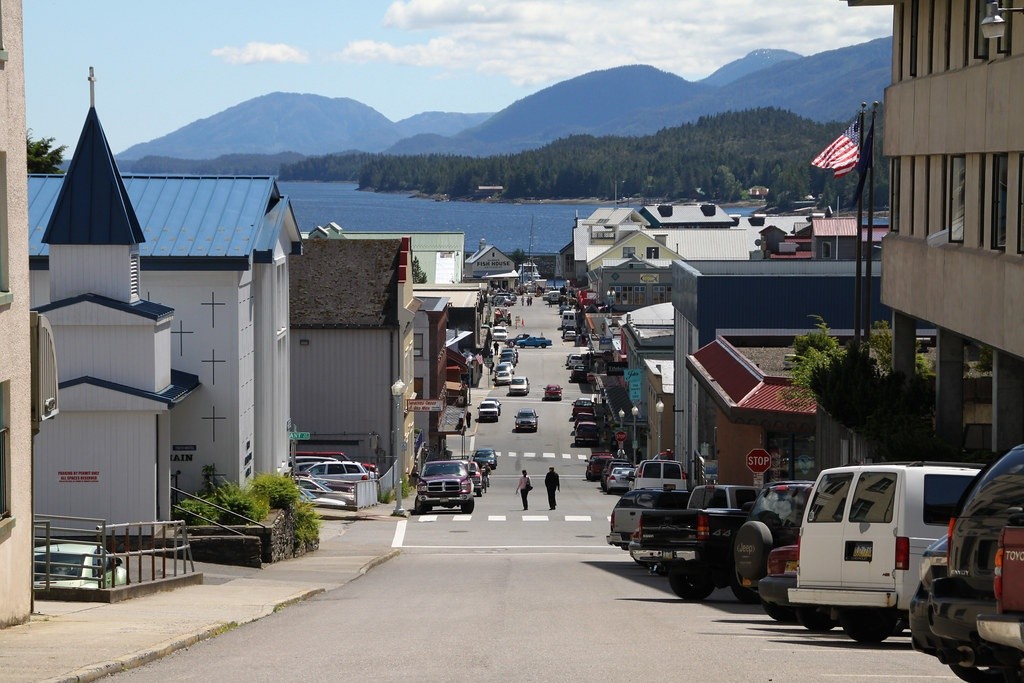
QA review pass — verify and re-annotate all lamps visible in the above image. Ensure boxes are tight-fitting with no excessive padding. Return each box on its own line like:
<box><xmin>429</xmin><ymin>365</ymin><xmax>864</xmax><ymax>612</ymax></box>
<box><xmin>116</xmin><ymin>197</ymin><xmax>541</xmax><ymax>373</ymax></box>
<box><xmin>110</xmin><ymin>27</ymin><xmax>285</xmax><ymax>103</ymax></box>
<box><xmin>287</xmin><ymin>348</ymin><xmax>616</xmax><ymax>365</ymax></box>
<box><xmin>981</xmin><ymin>2</ymin><xmax>1024</xmax><ymax>40</ymax></box>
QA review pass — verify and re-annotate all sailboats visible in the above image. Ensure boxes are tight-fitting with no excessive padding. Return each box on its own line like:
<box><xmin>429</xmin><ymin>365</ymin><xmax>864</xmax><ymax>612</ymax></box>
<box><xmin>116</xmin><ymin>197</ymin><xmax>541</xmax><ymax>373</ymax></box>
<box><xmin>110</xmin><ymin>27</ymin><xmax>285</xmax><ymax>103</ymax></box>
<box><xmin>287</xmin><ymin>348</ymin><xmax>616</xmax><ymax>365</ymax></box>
<box><xmin>511</xmin><ymin>214</ymin><xmax>547</xmax><ymax>290</ymax></box>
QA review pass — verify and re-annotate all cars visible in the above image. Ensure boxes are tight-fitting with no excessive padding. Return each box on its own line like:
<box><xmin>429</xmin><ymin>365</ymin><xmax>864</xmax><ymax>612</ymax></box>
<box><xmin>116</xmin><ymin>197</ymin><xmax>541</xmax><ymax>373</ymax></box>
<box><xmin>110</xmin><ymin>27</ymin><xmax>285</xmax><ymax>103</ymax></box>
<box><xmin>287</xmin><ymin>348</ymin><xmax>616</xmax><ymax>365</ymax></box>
<box><xmin>584</xmin><ymin>452</ymin><xmax>635</xmax><ymax>492</ymax></box>
<box><xmin>286</xmin><ymin>452</ymin><xmax>374</xmax><ymax>507</ymax></box>
<box><xmin>477</xmin><ymin>294</ymin><xmax>599</xmax><ymax>446</ymax></box>
<box><xmin>33</xmin><ymin>543</ymin><xmax>127</xmax><ymax>589</ymax></box>
<box><xmin>472</xmin><ymin>449</ymin><xmax>498</xmax><ymax>470</ymax></box>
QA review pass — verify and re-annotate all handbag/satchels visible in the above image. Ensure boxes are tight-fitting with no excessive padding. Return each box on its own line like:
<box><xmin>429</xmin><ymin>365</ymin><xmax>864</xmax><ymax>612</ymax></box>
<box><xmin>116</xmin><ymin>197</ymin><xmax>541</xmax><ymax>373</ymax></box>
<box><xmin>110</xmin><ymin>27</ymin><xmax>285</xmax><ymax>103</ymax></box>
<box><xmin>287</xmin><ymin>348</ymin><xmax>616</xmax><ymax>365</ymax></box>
<box><xmin>526</xmin><ymin>477</ymin><xmax>533</xmax><ymax>492</ymax></box>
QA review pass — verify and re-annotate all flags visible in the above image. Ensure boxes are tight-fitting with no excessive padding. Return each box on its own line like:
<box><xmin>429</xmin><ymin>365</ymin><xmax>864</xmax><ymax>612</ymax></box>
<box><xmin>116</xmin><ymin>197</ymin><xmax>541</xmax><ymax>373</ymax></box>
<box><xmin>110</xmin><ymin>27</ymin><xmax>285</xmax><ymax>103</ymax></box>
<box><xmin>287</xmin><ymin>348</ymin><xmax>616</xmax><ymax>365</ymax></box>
<box><xmin>811</xmin><ymin>111</ymin><xmax>874</xmax><ymax>212</ymax></box>
<box><xmin>475</xmin><ymin>351</ymin><xmax>484</xmax><ymax>365</ymax></box>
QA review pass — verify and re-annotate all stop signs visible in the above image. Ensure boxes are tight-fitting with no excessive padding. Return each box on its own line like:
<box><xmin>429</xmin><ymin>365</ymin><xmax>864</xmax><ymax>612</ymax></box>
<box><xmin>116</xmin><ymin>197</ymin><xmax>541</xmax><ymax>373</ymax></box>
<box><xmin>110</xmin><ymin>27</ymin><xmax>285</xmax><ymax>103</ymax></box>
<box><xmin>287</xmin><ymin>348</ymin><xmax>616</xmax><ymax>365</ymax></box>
<box><xmin>746</xmin><ymin>448</ymin><xmax>771</xmax><ymax>473</ymax></box>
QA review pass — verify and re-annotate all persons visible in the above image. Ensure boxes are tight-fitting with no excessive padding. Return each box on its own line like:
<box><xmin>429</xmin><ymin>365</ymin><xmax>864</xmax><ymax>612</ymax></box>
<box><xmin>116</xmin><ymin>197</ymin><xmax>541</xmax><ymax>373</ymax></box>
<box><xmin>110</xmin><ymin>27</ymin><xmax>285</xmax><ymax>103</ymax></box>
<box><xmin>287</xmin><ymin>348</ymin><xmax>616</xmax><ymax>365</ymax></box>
<box><xmin>548</xmin><ymin>296</ymin><xmax>553</xmax><ymax>308</ymax></box>
<box><xmin>545</xmin><ymin>467</ymin><xmax>560</xmax><ymax>510</ymax></box>
<box><xmin>515</xmin><ymin>470</ymin><xmax>531</xmax><ymax>510</ymax></box>
<box><xmin>542</xmin><ymin>287</ymin><xmax>545</xmax><ymax>294</ymax></box>
<box><xmin>490</xmin><ymin>360</ymin><xmax>495</xmax><ymax>375</ymax></box>
<box><xmin>493</xmin><ymin>341</ymin><xmax>499</xmax><ymax>355</ymax></box>
<box><xmin>520</xmin><ymin>295</ymin><xmax>532</xmax><ymax>306</ymax></box>
<box><xmin>481</xmin><ymin>463</ymin><xmax>491</xmax><ymax>488</ymax></box>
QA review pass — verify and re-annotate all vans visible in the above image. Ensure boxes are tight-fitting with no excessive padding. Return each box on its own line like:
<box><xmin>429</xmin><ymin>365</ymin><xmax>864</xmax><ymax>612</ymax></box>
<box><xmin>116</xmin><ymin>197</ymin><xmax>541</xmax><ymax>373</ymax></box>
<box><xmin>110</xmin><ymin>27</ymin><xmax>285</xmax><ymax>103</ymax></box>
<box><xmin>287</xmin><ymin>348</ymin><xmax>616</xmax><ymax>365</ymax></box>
<box><xmin>788</xmin><ymin>461</ymin><xmax>988</xmax><ymax>643</ymax></box>
<box><xmin>561</xmin><ymin>311</ymin><xmax>576</xmax><ymax>328</ymax></box>
<box><xmin>628</xmin><ymin>460</ymin><xmax>687</xmax><ymax>493</ymax></box>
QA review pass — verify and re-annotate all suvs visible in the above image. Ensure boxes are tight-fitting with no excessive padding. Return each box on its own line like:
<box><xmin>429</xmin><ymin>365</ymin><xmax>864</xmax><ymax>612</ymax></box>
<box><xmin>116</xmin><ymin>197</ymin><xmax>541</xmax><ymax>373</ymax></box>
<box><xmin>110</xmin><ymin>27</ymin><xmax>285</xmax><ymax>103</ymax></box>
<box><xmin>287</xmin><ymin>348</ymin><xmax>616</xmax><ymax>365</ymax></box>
<box><xmin>412</xmin><ymin>459</ymin><xmax>485</xmax><ymax>514</ymax></box>
<box><xmin>515</xmin><ymin>409</ymin><xmax>539</xmax><ymax>431</ymax></box>
<box><xmin>732</xmin><ymin>482</ymin><xmax>815</xmax><ymax>594</ymax></box>
<box><xmin>607</xmin><ymin>486</ymin><xmax>692</xmax><ymax>566</ymax></box>
<box><xmin>909</xmin><ymin>437</ymin><xmax>1024</xmax><ymax>683</ymax></box>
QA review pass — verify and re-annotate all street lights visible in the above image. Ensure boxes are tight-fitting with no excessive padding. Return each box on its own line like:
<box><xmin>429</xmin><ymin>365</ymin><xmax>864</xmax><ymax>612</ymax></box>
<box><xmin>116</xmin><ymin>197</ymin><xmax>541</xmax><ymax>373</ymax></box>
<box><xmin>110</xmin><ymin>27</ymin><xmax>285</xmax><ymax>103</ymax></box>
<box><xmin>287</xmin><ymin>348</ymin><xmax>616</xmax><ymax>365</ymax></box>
<box><xmin>391</xmin><ymin>380</ymin><xmax>405</xmax><ymax>515</ymax></box>
<box><xmin>619</xmin><ymin>405</ymin><xmax>639</xmax><ymax>466</ymax></box>
<box><xmin>656</xmin><ymin>400</ymin><xmax>664</xmax><ymax>460</ymax></box>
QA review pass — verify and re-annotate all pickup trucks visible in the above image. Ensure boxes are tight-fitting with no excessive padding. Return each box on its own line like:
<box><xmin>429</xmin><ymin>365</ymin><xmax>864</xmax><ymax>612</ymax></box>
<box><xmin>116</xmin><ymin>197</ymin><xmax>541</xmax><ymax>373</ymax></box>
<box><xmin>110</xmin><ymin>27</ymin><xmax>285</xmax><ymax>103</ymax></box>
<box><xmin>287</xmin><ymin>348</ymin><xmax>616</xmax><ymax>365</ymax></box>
<box><xmin>627</xmin><ymin>485</ymin><xmax>756</xmax><ymax>602</ymax></box>
<box><xmin>976</xmin><ymin>523</ymin><xmax>1024</xmax><ymax>649</ymax></box>
